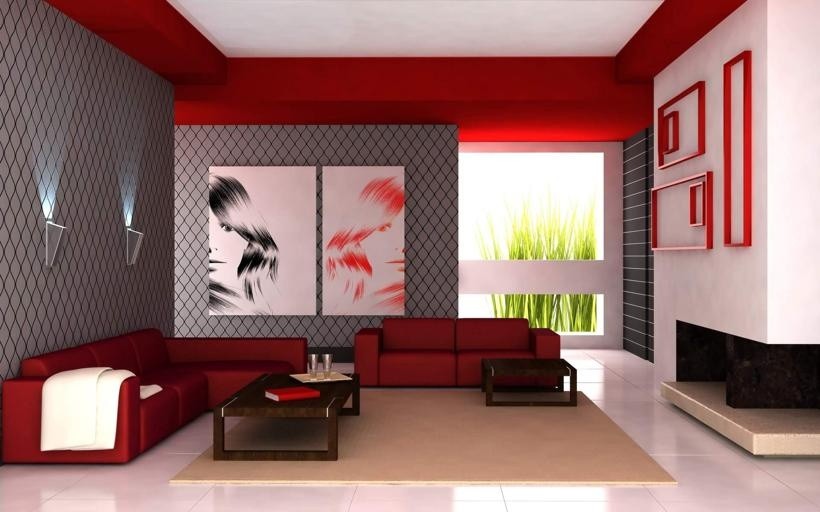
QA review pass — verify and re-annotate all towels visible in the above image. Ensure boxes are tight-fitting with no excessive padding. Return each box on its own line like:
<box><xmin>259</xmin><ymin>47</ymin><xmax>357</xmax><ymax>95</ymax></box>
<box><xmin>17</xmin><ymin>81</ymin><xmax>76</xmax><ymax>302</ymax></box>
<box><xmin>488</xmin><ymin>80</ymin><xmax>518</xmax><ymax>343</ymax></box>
<box><xmin>42</xmin><ymin>365</ymin><xmax>164</xmax><ymax>452</ymax></box>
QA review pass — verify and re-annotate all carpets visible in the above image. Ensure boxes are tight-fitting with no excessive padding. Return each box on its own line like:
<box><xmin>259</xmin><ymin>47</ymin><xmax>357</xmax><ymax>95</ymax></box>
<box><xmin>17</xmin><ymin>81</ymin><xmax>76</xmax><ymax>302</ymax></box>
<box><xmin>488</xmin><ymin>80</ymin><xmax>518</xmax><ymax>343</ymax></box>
<box><xmin>169</xmin><ymin>386</ymin><xmax>675</xmax><ymax>484</ymax></box>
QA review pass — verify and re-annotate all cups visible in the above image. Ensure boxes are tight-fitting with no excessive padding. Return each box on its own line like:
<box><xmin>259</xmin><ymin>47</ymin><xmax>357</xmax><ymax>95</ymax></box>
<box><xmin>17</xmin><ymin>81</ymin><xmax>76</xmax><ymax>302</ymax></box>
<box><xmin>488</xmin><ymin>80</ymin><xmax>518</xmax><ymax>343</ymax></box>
<box><xmin>321</xmin><ymin>353</ymin><xmax>334</xmax><ymax>380</ymax></box>
<box><xmin>308</xmin><ymin>354</ymin><xmax>319</xmax><ymax>380</ymax></box>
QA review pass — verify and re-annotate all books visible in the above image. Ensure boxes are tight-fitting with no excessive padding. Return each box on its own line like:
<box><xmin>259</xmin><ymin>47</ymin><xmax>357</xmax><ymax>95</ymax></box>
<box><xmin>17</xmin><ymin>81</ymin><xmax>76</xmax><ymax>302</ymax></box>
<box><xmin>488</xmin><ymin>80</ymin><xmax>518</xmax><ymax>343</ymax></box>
<box><xmin>264</xmin><ymin>385</ymin><xmax>321</xmax><ymax>402</ymax></box>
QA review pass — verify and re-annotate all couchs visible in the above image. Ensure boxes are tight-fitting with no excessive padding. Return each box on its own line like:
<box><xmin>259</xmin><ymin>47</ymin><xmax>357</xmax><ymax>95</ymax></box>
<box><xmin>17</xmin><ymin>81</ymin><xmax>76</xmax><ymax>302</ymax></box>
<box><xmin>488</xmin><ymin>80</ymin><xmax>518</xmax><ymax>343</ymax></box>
<box><xmin>2</xmin><ymin>327</ymin><xmax>309</xmax><ymax>466</ymax></box>
<box><xmin>353</xmin><ymin>318</ymin><xmax>563</xmax><ymax>391</ymax></box>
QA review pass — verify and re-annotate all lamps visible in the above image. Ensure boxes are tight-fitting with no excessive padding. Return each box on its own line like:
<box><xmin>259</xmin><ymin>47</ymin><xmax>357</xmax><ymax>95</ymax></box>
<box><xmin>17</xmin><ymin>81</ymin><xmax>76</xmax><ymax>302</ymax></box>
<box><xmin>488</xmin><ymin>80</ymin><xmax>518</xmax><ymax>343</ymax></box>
<box><xmin>44</xmin><ymin>220</ymin><xmax>66</xmax><ymax>269</ymax></box>
<box><xmin>126</xmin><ymin>229</ymin><xmax>144</xmax><ymax>265</ymax></box>
